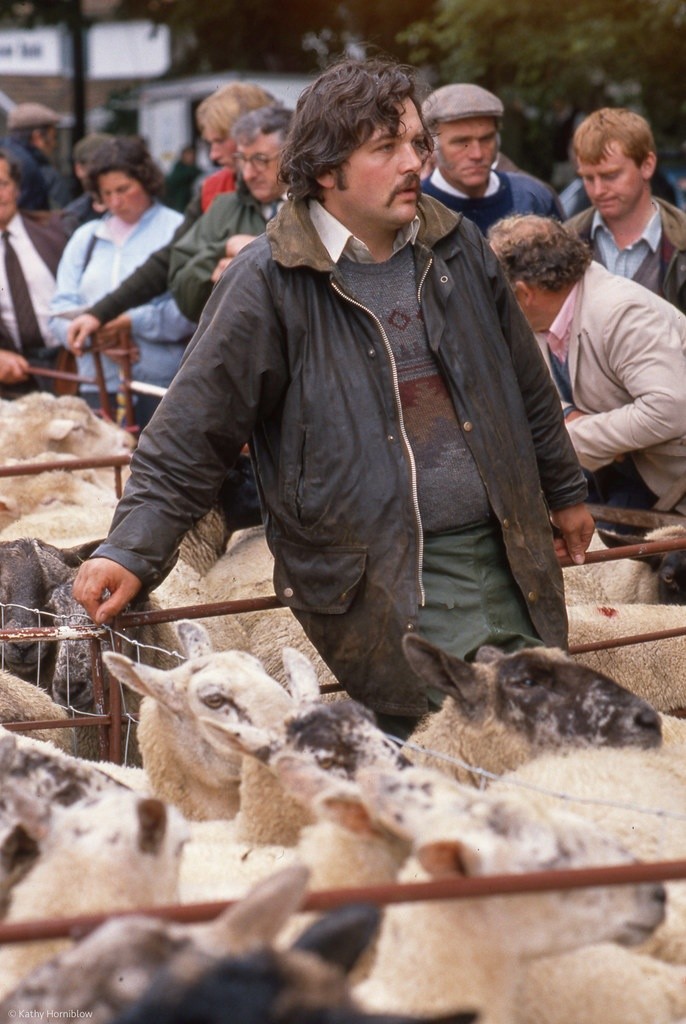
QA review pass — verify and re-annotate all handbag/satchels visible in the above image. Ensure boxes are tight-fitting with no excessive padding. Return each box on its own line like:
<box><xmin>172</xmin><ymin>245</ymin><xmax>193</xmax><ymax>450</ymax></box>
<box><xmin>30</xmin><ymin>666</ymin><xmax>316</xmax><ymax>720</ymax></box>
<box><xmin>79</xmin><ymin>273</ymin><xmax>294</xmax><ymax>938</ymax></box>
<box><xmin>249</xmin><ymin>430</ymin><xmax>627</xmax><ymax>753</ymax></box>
<box><xmin>53</xmin><ymin>348</ymin><xmax>79</xmax><ymax>396</ymax></box>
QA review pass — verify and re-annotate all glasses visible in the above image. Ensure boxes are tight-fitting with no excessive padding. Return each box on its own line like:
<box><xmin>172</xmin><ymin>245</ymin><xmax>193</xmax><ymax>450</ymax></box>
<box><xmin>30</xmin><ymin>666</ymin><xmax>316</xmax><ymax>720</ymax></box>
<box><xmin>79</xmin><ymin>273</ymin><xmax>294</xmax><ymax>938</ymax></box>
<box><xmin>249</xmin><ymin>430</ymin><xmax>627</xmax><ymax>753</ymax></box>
<box><xmin>231</xmin><ymin>146</ymin><xmax>285</xmax><ymax>172</ymax></box>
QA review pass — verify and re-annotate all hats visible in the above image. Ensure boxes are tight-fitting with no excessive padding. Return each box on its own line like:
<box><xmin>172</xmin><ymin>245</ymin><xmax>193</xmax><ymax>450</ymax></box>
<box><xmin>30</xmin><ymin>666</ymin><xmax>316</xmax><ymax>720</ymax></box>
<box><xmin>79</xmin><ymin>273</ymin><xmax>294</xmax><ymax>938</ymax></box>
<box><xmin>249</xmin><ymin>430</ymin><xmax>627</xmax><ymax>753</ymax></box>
<box><xmin>7</xmin><ymin>102</ymin><xmax>62</xmax><ymax>130</ymax></box>
<box><xmin>422</xmin><ymin>83</ymin><xmax>504</xmax><ymax>127</ymax></box>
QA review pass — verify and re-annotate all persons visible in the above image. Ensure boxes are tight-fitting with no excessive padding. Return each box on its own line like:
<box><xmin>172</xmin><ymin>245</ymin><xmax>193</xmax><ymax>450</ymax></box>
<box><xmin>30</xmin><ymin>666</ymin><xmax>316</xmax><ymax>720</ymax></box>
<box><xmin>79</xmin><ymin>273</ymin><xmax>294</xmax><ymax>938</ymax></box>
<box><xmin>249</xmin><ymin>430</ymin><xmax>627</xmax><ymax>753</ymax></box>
<box><xmin>66</xmin><ymin>56</ymin><xmax>597</xmax><ymax>732</ymax></box>
<box><xmin>1</xmin><ymin>96</ymin><xmax>64</xmax><ymax>213</ymax></box>
<box><xmin>169</xmin><ymin>101</ymin><xmax>296</xmax><ymax>325</ymax></box>
<box><xmin>52</xmin><ymin>133</ymin><xmax>116</xmax><ymax>238</ymax></box>
<box><xmin>0</xmin><ymin>149</ymin><xmax>75</xmax><ymax>396</ymax></box>
<box><xmin>47</xmin><ymin>131</ymin><xmax>187</xmax><ymax>426</ymax></box>
<box><xmin>67</xmin><ymin>80</ymin><xmax>288</xmax><ymax>381</ymax></box>
<box><xmin>483</xmin><ymin>211</ymin><xmax>686</xmax><ymax>578</ymax></box>
<box><xmin>545</xmin><ymin>106</ymin><xmax>686</xmax><ymax>308</ymax></box>
<box><xmin>409</xmin><ymin>80</ymin><xmax>566</xmax><ymax>229</ymax></box>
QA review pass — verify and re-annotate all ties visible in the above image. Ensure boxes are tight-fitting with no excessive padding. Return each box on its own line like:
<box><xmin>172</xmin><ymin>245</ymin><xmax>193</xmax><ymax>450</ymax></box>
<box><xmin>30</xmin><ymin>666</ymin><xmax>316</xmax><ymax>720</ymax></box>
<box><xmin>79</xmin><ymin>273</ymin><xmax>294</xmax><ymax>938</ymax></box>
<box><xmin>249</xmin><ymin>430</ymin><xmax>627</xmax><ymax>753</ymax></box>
<box><xmin>1</xmin><ymin>231</ymin><xmax>47</xmax><ymax>359</ymax></box>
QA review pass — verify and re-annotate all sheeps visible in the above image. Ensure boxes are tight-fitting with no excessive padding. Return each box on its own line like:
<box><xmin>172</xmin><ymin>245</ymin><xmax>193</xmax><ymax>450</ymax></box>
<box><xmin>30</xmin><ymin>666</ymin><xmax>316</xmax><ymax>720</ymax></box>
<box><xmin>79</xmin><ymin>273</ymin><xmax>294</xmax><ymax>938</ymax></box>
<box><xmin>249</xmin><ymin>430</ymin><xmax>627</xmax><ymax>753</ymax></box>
<box><xmin>0</xmin><ymin>387</ymin><xmax>686</xmax><ymax>1023</ymax></box>
